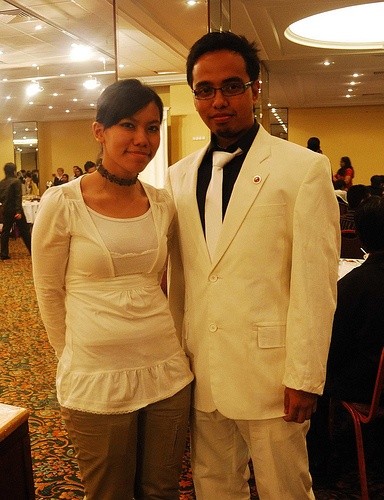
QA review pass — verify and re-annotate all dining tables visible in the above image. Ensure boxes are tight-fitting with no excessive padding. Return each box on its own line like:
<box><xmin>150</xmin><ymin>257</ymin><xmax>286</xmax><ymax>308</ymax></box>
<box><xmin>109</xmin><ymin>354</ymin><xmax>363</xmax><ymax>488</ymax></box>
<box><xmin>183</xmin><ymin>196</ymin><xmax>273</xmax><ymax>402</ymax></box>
<box><xmin>0</xmin><ymin>200</ymin><xmax>39</xmax><ymax>240</ymax></box>
<box><xmin>336</xmin><ymin>258</ymin><xmax>366</xmax><ymax>280</ymax></box>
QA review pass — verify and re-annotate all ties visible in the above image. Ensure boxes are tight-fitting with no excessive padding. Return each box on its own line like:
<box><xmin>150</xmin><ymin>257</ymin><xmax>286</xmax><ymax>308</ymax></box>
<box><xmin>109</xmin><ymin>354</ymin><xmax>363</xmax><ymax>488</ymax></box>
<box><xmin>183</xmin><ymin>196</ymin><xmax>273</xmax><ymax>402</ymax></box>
<box><xmin>205</xmin><ymin>147</ymin><xmax>243</xmax><ymax>264</ymax></box>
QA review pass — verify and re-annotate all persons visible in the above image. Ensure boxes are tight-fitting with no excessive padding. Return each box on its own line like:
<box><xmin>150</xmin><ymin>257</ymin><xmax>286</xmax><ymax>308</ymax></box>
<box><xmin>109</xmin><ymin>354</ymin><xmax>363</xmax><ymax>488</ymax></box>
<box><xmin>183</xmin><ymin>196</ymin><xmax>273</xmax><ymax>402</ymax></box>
<box><xmin>31</xmin><ymin>78</ymin><xmax>194</xmax><ymax>500</ymax></box>
<box><xmin>164</xmin><ymin>30</ymin><xmax>342</xmax><ymax>500</ymax></box>
<box><xmin>0</xmin><ymin>163</ymin><xmax>32</xmax><ymax>260</ymax></box>
<box><xmin>17</xmin><ymin>168</ymin><xmax>40</xmax><ymax>197</ymax></box>
<box><xmin>333</xmin><ymin>156</ymin><xmax>355</xmax><ymax>192</ymax></box>
<box><xmin>46</xmin><ymin>160</ymin><xmax>97</xmax><ymax>188</ymax></box>
<box><xmin>315</xmin><ymin>174</ymin><xmax>384</xmax><ymax>483</ymax></box>
<box><xmin>306</xmin><ymin>136</ymin><xmax>322</xmax><ymax>154</ymax></box>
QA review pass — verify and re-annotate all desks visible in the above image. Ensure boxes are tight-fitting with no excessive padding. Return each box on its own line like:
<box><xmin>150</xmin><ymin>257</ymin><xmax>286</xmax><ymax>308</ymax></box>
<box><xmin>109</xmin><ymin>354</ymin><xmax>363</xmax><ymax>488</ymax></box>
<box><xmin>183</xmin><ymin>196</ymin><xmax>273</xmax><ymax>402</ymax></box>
<box><xmin>0</xmin><ymin>403</ymin><xmax>35</xmax><ymax>500</ymax></box>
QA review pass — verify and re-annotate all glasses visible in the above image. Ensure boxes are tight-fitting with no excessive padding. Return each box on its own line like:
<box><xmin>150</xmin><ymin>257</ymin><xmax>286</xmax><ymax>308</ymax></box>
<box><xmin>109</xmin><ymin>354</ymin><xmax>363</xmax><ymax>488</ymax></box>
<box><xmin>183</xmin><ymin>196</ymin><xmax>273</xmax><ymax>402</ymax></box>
<box><xmin>192</xmin><ymin>81</ymin><xmax>253</xmax><ymax>100</ymax></box>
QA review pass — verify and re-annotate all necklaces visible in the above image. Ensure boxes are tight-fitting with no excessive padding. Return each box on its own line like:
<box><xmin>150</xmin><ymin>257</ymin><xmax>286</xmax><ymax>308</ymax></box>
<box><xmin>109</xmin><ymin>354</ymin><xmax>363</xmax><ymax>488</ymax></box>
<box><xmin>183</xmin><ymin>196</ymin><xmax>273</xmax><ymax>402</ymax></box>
<box><xmin>98</xmin><ymin>163</ymin><xmax>139</xmax><ymax>186</ymax></box>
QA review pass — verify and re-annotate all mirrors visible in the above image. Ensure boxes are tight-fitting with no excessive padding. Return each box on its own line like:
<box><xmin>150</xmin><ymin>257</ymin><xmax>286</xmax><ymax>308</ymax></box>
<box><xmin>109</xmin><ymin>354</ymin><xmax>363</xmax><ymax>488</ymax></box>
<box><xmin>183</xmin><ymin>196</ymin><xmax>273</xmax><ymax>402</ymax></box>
<box><xmin>11</xmin><ymin>121</ymin><xmax>39</xmax><ymax>187</ymax></box>
<box><xmin>255</xmin><ymin>106</ymin><xmax>289</xmax><ymax>141</ymax></box>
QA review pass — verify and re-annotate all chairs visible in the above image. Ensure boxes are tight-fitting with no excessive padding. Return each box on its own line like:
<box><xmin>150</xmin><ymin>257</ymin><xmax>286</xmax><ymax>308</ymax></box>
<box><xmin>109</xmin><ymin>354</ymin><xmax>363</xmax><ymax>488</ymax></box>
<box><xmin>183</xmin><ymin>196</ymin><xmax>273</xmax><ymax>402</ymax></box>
<box><xmin>340</xmin><ymin>229</ymin><xmax>365</xmax><ymax>259</ymax></box>
<box><xmin>327</xmin><ymin>348</ymin><xmax>384</xmax><ymax>500</ymax></box>
